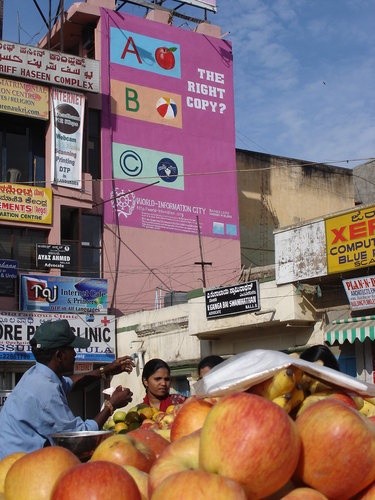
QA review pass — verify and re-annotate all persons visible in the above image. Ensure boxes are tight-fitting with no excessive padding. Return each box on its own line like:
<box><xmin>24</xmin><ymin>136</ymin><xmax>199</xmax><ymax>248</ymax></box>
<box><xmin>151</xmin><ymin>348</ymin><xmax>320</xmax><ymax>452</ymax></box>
<box><xmin>197</xmin><ymin>356</ymin><xmax>223</xmax><ymax>380</ymax></box>
<box><xmin>0</xmin><ymin>319</ymin><xmax>136</xmax><ymax>458</ymax></box>
<box><xmin>142</xmin><ymin>358</ymin><xmax>187</xmax><ymax>412</ymax></box>
<box><xmin>299</xmin><ymin>345</ymin><xmax>338</xmax><ymax>371</ymax></box>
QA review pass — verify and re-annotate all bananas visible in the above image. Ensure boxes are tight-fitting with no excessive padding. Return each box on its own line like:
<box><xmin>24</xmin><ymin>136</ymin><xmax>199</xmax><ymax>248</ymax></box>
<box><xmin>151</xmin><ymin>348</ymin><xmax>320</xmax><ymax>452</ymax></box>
<box><xmin>247</xmin><ymin>351</ymin><xmax>375</xmax><ymax>417</ymax></box>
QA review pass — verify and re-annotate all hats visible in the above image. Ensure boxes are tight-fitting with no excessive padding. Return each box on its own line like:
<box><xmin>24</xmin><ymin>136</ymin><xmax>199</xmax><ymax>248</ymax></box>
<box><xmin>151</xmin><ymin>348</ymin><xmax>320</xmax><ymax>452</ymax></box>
<box><xmin>31</xmin><ymin>318</ymin><xmax>90</xmax><ymax>352</ymax></box>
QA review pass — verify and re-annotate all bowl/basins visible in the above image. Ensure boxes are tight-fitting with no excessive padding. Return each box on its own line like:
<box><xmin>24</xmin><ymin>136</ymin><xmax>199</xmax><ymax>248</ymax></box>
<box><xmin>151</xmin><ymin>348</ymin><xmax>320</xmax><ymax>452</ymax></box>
<box><xmin>47</xmin><ymin>430</ymin><xmax>114</xmax><ymax>463</ymax></box>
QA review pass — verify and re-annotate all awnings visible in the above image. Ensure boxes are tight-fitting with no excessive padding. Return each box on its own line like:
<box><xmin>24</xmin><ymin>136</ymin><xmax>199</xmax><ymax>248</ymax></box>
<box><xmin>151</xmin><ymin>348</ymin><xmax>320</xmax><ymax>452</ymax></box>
<box><xmin>324</xmin><ymin>316</ymin><xmax>375</xmax><ymax>347</ymax></box>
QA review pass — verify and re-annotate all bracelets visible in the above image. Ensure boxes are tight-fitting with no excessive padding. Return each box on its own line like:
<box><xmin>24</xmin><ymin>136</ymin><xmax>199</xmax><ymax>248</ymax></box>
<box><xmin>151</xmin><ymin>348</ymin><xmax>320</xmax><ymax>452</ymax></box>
<box><xmin>103</xmin><ymin>401</ymin><xmax>114</xmax><ymax>416</ymax></box>
<box><xmin>100</xmin><ymin>366</ymin><xmax>106</xmax><ymax>380</ymax></box>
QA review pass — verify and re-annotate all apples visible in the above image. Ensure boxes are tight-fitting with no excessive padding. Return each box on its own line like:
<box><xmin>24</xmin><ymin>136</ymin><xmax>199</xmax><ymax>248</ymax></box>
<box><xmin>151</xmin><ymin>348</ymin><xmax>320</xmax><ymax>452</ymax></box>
<box><xmin>1</xmin><ymin>392</ymin><xmax>375</xmax><ymax>500</ymax></box>
